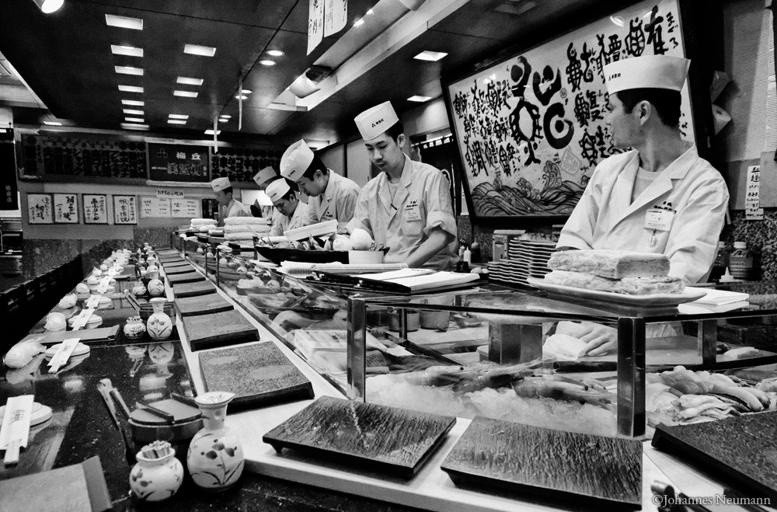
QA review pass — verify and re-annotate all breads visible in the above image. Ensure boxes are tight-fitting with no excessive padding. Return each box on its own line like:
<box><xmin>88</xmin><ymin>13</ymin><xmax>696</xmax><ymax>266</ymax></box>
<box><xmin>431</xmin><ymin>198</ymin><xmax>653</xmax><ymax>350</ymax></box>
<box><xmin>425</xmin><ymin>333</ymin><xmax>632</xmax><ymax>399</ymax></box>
<box><xmin>223</xmin><ymin>217</ymin><xmax>273</xmax><ymax>241</ymax></box>
<box><xmin>539</xmin><ymin>250</ymin><xmax>684</xmax><ymax>296</ymax></box>
<box><xmin>546</xmin><ymin>333</ymin><xmax>589</xmax><ymax>360</ymax></box>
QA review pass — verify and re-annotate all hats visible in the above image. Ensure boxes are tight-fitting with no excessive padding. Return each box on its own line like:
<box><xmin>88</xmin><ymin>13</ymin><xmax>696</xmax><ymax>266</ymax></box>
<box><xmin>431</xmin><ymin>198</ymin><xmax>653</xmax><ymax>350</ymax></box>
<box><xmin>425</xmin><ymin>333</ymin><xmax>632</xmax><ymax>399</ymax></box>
<box><xmin>266</xmin><ymin>178</ymin><xmax>290</xmax><ymax>202</ymax></box>
<box><xmin>252</xmin><ymin>166</ymin><xmax>277</xmax><ymax>186</ymax></box>
<box><xmin>211</xmin><ymin>177</ymin><xmax>232</xmax><ymax>192</ymax></box>
<box><xmin>279</xmin><ymin>139</ymin><xmax>314</xmax><ymax>182</ymax></box>
<box><xmin>354</xmin><ymin>100</ymin><xmax>399</xmax><ymax>142</ymax></box>
<box><xmin>602</xmin><ymin>55</ymin><xmax>692</xmax><ymax>97</ymax></box>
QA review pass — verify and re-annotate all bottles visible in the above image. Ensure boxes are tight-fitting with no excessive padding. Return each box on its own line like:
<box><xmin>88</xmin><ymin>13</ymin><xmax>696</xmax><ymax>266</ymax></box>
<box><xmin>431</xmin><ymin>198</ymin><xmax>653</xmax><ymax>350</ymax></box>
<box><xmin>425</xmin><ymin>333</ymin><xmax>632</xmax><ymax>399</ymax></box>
<box><xmin>143</xmin><ymin>243</ymin><xmax>164</xmax><ymax>296</ymax></box>
<box><xmin>185</xmin><ymin>390</ymin><xmax>246</xmax><ymax>490</ymax></box>
<box><xmin>146</xmin><ymin>297</ymin><xmax>173</xmax><ymax>341</ymax></box>
<box><xmin>148</xmin><ymin>342</ymin><xmax>176</xmax><ymax>380</ymax></box>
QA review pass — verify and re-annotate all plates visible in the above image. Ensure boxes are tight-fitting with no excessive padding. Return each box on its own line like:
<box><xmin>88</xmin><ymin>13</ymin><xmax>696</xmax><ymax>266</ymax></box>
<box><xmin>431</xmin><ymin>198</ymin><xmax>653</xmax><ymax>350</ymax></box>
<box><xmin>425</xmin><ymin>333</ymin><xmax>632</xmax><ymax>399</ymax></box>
<box><xmin>487</xmin><ymin>236</ymin><xmax>556</xmax><ymax>288</ymax></box>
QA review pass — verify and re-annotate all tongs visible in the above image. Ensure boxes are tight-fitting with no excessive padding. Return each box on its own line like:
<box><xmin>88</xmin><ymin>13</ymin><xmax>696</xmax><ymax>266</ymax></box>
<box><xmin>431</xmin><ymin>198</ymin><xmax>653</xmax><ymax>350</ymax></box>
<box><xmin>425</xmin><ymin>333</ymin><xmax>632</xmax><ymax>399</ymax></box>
<box><xmin>95</xmin><ymin>376</ymin><xmax>137</xmax><ymax>470</ymax></box>
<box><xmin>129</xmin><ymin>357</ymin><xmax>144</xmax><ymax>378</ymax></box>
<box><xmin>125</xmin><ymin>290</ymin><xmax>141</xmax><ymax>312</ymax></box>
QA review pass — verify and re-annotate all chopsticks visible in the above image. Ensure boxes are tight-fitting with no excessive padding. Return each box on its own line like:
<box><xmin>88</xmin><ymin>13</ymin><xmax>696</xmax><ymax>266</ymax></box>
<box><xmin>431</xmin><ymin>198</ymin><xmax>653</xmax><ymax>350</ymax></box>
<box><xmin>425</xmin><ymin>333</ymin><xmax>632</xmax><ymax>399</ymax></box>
<box><xmin>2</xmin><ymin>447</ymin><xmax>22</xmax><ymax>465</ymax></box>
<box><xmin>47</xmin><ymin>365</ymin><xmax>60</xmax><ymax>374</ymax></box>
<box><xmin>71</xmin><ymin>326</ymin><xmax>81</xmax><ymax>332</ymax></box>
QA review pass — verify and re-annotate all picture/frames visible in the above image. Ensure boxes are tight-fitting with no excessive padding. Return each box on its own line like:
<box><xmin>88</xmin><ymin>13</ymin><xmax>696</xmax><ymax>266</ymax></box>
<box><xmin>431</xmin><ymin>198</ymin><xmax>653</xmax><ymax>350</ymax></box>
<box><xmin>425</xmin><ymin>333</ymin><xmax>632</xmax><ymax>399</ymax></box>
<box><xmin>52</xmin><ymin>192</ymin><xmax>78</xmax><ymax>224</ymax></box>
<box><xmin>26</xmin><ymin>192</ymin><xmax>53</xmax><ymax>225</ymax></box>
<box><xmin>81</xmin><ymin>192</ymin><xmax>107</xmax><ymax>224</ymax></box>
<box><xmin>441</xmin><ymin>0</ymin><xmax>730</xmax><ymax>226</ymax></box>
<box><xmin>112</xmin><ymin>194</ymin><xmax>137</xmax><ymax>226</ymax></box>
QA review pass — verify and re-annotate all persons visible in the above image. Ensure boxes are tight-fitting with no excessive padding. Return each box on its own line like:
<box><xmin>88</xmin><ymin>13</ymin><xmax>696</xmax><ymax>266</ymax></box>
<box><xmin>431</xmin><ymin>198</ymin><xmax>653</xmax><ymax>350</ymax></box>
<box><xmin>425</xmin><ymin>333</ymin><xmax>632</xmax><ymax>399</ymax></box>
<box><xmin>266</xmin><ymin>177</ymin><xmax>309</xmax><ymax>237</ymax></box>
<box><xmin>211</xmin><ymin>175</ymin><xmax>251</xmax><ymax>228</ymax></box>
<box><xmin>278</xmin><ymin>138</ymin><xmax>363</xmax><ymax>236</ymax></box>
<box><xmin>554</xmin><ymin>54</ymin><xmax>731</xmax><ymax>358</ymax></box>
<box><xmin>254</xmin><ymin>165</ymin><xmax>309</xmax><ymax>223</ymax></box>
<box><xmin>347</xmin><ymin>99</ymin><xmax>461</xmax><ymax>268</ymax></box>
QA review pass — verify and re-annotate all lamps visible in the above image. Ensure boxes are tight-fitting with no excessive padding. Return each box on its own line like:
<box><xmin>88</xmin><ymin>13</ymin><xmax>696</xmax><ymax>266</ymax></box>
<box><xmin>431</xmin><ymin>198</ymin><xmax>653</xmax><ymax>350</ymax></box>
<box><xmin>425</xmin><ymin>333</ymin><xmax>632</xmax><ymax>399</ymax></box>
<box><xmin>34</xmin><ymin>1</ymin><xmax>66</xmax><ymax>16</ymax></box>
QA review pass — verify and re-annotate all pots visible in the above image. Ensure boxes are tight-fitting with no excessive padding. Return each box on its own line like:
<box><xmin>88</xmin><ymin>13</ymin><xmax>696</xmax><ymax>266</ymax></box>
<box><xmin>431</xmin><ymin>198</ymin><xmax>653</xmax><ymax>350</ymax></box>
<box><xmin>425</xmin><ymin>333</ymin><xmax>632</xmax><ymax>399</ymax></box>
<box><xmin>126</xmin><ymin>413</ymin><xmax>203</xmax><ymax>444</ymax></box>
